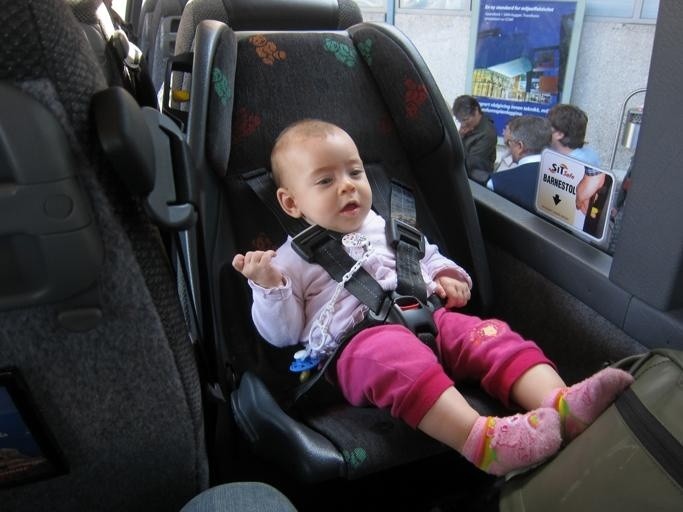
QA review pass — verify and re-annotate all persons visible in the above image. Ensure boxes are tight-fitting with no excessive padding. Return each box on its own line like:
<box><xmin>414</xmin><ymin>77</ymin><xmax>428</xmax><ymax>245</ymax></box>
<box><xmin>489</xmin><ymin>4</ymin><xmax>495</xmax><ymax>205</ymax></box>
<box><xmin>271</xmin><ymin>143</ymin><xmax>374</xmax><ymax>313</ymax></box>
<box><xmin>548</xmin><ymin>104</ymin><xmax>601</xmax><ymax>168</ymax></box>
<box><xmin>231</xmin><ymin>115</ymin><xmax>636</xmax><ymax>476</ymax></box>
<box><xmin>575</xmin><ymin>166</ymin><xmax>606</xmax><ymax>214</ymax></box>
<box><xmin>486</xmin><ymin>114</ymin><xmax>554</xmax><ymax>213</ymax></box>
<box><xmin>452</xmin><ymin>95</ymin><xmax>497</xmax><ymax>186</ymax></box>
